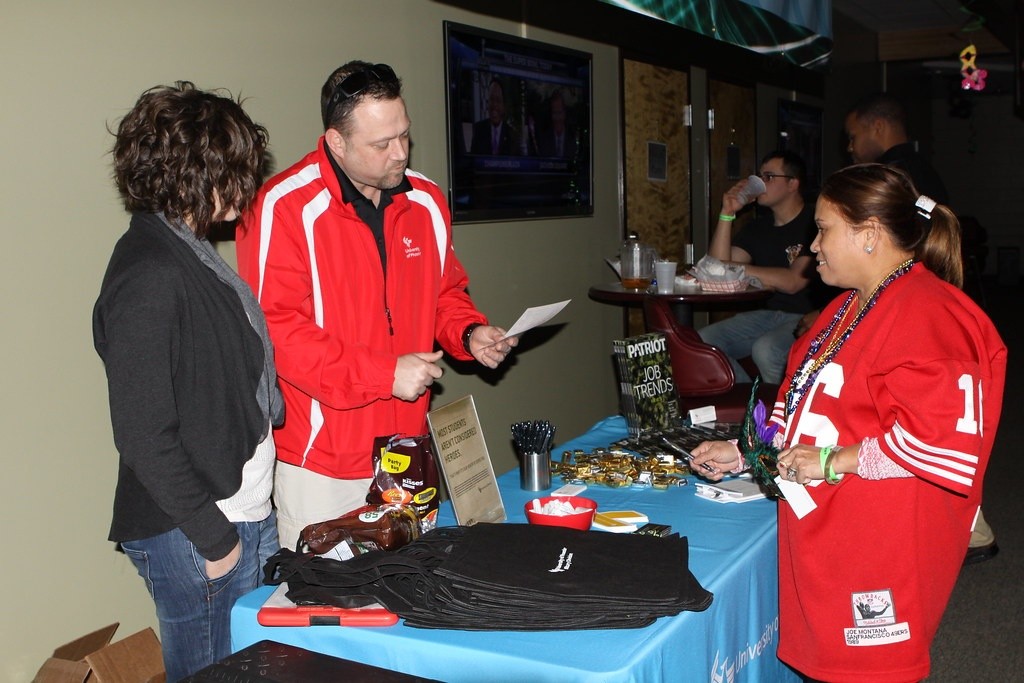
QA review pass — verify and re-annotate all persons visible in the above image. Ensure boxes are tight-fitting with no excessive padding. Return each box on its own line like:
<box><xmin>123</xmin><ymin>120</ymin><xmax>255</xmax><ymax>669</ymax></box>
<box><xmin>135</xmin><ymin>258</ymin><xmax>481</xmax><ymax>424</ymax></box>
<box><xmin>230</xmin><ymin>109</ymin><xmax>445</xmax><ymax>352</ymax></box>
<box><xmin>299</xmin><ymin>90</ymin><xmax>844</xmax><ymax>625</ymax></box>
<box><xmin>235</xmin><ymin>60</ymin><xmax>519</xmax><ymax>552</ymax></box>
<box><xmin>695</xmin><ymin>148</ymin><xmax>838</xmax><ymax>385</ymax></box>
<box><xmin>470</xmin><ymin>74</ymin><xmax>520</xmax><ymax>156</ymax></box>
<box><xmin>538</xmin><ymin>89</ymin><xmax>576</xmax><ymax>157</ymax></box>
<box><xmin>796</xmin><ymin>93</ymin><xmax>1000</xmax><ymax>566</ymax></box>
<box><xmin>92</xmin><ymin>81</ymin><xmax>285</xmax><ymax>683</ymax></box>
<box><xmin>689</xmin><ymin>162</ymin><xmax>1008</xmax><ymax>683</ymax></box>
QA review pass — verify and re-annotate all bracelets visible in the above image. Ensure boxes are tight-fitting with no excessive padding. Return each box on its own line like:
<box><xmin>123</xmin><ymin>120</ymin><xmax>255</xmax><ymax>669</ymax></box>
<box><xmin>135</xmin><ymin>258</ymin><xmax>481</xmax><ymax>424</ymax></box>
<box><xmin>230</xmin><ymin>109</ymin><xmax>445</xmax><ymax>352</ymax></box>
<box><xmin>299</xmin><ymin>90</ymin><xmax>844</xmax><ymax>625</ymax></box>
<box><xmin>719</xmin><ymin>214</ymin><xmax>736</xmax><ymax>221</ymax></box>
<box><xmin>819</xmin><ymin>444</ymin><xmax>845</xmax><ymax>484</ymax></box>
<box><xmin>461</xmin><ymin>323</ymin><xmax>482</xmax><ymax>353</ymax></box>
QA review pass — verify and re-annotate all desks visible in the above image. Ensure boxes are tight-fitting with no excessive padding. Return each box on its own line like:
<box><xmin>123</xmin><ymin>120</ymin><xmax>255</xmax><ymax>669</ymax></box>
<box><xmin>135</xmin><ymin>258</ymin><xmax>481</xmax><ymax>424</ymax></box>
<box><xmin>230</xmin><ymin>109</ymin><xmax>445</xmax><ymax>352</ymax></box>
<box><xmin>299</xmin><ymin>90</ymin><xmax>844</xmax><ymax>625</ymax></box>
<box><xmin>229</xmin><ymin>413</ymin><xmax>783</xmax><ymax>683</ymax></box>
<box><xmin>588</xmin><ymin>279</ymin><xmax>776</xmax><ymax>340</ymax></box>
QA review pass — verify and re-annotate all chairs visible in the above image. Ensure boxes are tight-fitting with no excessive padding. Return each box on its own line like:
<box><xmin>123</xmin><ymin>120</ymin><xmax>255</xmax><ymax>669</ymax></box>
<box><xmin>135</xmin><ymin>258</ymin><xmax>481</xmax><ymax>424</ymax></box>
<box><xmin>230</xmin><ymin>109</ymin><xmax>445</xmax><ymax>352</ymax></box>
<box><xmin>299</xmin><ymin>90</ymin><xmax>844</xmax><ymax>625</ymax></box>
<box><xmin>644</xmin><ymin>295</ymin><xmax>757</xmax><ymax>424</ymax></box>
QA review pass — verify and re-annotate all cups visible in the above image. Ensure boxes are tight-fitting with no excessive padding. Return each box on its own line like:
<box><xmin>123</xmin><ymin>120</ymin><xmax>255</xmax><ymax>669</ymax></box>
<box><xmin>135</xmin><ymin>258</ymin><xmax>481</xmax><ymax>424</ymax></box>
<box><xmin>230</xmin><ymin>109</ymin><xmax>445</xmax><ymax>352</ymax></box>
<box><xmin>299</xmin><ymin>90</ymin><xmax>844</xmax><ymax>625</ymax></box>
<box><xmin>524</xmin><ymin>456</ymin><xmax>549</xmax><ymax>493</ymax></box>
<box><xmin>653</xmin><ymin>262</ymin><xmax>677</xmax><ymax>295</ymax></box>
<box><xmin>737</xmin><ymin>174</ymin><xmax>767</xmax><ymax>204</ymax></box>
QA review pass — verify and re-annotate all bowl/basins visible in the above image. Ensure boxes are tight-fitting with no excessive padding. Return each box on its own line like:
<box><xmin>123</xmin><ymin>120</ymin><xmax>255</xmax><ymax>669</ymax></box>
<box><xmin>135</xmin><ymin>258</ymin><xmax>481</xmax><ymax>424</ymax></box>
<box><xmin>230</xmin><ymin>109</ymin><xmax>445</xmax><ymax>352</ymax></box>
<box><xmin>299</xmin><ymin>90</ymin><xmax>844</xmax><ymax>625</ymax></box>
<box><xmin>525</xmin><ymin>495</ymin><xmax>597</xmax><ymax>534</ymax></box>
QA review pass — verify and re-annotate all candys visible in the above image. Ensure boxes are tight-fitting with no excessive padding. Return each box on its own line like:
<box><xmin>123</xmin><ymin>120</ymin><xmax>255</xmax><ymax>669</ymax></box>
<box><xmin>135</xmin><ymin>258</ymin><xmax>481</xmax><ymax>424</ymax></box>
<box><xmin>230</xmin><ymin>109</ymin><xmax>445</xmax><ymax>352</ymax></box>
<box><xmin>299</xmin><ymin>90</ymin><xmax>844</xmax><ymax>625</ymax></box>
<box><xmin>550</xmin><ymin>445</ymin><xmax>693</xmax><ymax>490</ymax></box>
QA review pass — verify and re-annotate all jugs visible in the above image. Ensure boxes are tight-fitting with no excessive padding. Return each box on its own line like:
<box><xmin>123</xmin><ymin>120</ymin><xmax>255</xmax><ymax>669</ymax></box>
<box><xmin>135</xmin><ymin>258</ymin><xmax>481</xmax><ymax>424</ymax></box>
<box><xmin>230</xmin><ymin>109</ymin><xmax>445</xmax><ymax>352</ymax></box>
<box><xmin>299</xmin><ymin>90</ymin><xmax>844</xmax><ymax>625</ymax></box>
<box><xmin>620</xmin><ymin>235</ymin><xmax>652</xmax><ymax>289</ymax></box>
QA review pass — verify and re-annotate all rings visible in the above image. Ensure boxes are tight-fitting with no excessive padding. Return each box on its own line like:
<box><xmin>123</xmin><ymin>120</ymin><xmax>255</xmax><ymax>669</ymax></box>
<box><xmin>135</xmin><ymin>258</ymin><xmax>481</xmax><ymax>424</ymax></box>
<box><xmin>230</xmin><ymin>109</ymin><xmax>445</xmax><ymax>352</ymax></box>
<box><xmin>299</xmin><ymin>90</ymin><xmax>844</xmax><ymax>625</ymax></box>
<box><xmin>780</xmin><ymin>461</ymin><xmax>787</xmax><ymax>469</ymax></box>
<box><xmin>787</xmin><ymin>467</ymin><xmax>796</xmax><ymax>478</ymax></box>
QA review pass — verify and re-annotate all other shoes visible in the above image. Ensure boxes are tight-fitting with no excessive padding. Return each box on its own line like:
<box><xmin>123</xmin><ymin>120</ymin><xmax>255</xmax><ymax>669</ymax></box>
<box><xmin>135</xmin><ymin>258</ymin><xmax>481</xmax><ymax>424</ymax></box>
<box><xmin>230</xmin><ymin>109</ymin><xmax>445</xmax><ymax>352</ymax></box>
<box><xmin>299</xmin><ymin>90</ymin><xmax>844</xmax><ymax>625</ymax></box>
<box><xmin>963</xmin><ymin>541</ymin><xmax>999</xmax><ymax>567</ymax></box>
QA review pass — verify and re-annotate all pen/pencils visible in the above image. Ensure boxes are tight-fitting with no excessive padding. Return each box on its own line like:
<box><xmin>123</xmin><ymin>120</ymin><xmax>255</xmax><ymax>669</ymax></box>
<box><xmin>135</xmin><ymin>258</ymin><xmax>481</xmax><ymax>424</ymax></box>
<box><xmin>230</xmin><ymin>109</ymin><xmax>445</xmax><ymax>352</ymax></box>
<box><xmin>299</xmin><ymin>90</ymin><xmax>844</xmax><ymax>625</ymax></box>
<box><xmin>663</xmin><ymin>437</ymin><xmax>715</xmax><ymax>473</ymax></box>
<box><xmin>511</xmin><ymin>419</ymin><xmax>556</xmax><ymax>454</ymax></box>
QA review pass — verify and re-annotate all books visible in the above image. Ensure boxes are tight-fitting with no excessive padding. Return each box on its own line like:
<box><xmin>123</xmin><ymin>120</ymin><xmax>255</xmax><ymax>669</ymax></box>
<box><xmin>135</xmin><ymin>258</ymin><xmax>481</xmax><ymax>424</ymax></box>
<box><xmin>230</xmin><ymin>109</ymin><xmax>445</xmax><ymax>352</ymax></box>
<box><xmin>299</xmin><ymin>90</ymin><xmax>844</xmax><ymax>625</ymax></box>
<box><xmin>612</xmin><ymin>332</ymin><xmax>683</xmax><ymax>439</ymax></box>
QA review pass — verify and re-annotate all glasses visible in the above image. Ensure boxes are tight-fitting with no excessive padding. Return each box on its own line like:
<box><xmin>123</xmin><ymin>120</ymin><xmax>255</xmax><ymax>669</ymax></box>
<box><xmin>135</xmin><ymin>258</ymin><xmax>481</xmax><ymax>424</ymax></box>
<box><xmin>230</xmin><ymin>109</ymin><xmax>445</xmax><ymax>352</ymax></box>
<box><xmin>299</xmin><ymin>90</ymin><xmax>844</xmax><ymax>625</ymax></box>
<box><xmin>759</xmin><ymin>174</ymin><xmax>794</xmax><ymax>182</ymax></box>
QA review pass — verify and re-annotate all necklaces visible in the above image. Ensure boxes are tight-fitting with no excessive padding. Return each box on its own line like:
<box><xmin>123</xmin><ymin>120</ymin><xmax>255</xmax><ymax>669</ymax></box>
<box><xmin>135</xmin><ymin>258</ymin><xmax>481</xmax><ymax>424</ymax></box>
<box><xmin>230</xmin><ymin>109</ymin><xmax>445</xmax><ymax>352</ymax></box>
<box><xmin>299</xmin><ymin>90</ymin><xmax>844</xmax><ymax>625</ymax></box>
<box><xmin>785</xmin><ymin>259</ymin><xmax>915</xmax><ymax>414</ymax></box>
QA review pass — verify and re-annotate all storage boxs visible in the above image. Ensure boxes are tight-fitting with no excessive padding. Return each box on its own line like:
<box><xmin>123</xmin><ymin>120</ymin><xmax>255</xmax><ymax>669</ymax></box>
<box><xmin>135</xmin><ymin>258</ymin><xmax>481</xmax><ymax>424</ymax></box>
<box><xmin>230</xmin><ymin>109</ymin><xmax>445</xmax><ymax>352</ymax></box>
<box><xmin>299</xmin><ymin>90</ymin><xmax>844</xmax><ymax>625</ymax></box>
<box><xmin>31</xmin><ymin>621</ymin><xmax>167</xmax><ymax>683</ymax></box>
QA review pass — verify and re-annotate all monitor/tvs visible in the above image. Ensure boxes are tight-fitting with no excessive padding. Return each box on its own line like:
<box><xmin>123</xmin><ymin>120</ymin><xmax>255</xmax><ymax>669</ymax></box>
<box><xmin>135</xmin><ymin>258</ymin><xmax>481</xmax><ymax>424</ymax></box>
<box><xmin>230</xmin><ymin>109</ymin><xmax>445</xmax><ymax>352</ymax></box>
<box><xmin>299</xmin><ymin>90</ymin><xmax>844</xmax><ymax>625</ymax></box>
<box><xmin>443</xmin><ymin>19</ymin><xmax>594</xmax><ymax>226</ymax></box>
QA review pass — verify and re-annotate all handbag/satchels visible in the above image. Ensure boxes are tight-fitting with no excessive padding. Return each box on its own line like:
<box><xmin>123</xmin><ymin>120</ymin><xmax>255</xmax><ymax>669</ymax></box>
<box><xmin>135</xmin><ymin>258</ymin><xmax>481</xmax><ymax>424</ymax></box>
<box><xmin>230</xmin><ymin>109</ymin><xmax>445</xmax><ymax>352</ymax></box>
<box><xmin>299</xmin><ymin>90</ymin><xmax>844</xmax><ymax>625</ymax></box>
<box><xmin>262</xmin><ymin>521</ymin><xmax>715</xmax><ymax>632</ymax></box>
